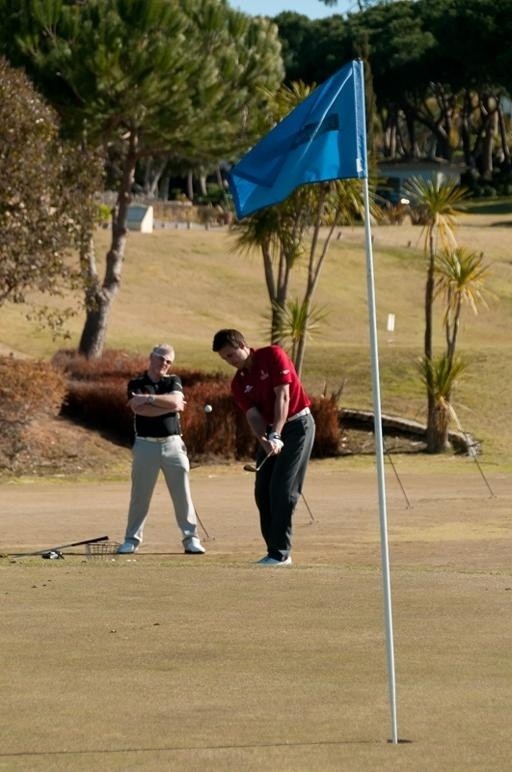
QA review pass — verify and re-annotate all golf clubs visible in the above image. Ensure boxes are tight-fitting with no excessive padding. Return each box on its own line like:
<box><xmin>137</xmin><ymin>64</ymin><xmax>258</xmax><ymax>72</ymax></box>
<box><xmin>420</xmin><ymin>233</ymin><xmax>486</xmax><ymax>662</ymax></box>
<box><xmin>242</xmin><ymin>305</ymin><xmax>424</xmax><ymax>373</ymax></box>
<box><xmin>244</xmin><ymin>450</ymin><xmax>274</xmax><ymax>471</ymax></box>
<box><xmin>8</xmin><ymin>536</ymin><xmax>108</xmax><ymax>563</ymax></box>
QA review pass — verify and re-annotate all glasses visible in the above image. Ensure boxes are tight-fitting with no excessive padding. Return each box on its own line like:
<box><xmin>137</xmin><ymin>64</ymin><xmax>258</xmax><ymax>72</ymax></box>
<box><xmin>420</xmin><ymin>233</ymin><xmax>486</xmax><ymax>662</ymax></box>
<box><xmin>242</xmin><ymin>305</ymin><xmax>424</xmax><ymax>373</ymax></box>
<box><xmin>151</xmin><ymin>350</ymin><xmax>174</xmax><ymax>366</ymax></box>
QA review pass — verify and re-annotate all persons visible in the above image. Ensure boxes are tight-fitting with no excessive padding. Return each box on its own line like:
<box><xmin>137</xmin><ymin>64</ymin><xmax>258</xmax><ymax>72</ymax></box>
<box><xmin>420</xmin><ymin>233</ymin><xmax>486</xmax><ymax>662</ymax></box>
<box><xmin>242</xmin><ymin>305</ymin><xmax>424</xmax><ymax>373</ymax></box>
<box><xmin>115</xmin><ymin>344</ymin><xmax>205</xmax><ymax>554</ymax></box>
<box><xmin>211</xmin><ymin>329</ymin><xmax>317</xmax><ymax>565</ymax></box>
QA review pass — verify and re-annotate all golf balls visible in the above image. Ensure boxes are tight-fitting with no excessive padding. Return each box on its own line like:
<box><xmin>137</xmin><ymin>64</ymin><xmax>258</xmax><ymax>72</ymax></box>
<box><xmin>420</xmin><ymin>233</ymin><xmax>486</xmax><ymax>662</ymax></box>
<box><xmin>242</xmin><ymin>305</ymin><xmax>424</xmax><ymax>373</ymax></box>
<box><xmin>205</xmin><ymin>405</ymin><xmax>213</xmax><ymax>413</ymax></box>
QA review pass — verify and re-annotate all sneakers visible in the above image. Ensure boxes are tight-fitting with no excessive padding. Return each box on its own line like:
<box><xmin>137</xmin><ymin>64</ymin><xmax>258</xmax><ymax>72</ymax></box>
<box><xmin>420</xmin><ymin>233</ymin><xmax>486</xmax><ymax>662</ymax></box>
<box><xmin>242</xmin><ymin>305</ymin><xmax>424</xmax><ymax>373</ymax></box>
<box><xmin>183</xmin><ymin>542</ymin><xmax>205</xmax><ymax>554</ymax></box>
<box><xmin>257</xmin><ymin>555</ymin><xmax>292</xmax><ymax>566</ymax></box>
<box><xmin>117</xmin><ymin>541</ymin><xmax>140</xmax><ymax>554</ymax></box>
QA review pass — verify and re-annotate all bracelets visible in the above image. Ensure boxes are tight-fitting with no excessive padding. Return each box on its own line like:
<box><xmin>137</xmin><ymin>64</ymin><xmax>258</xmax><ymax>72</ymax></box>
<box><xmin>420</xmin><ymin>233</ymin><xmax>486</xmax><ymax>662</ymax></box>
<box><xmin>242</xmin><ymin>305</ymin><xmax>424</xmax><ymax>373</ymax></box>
<box><xmin>146</xmin><ymin>395</ymin><xmax>155</xmax><ymax>405</ymax></box>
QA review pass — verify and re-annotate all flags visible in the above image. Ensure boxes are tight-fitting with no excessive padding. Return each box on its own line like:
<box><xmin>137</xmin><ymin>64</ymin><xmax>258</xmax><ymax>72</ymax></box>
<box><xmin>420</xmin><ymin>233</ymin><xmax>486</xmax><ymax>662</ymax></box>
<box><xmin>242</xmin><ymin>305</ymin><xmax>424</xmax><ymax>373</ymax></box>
<box><xmin>226</xmin><ymin>59</ymin><xmax>364</xmax><ymax>221</ymax></box>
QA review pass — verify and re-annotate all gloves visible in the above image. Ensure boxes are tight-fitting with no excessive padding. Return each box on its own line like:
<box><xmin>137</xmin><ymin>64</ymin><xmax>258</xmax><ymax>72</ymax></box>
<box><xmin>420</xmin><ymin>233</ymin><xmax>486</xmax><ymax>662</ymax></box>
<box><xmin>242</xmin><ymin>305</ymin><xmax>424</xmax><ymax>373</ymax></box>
<box><xmin>127</xmin><ymin>392</ymin><xmax>156</xmax><ymax>410</ymax></box>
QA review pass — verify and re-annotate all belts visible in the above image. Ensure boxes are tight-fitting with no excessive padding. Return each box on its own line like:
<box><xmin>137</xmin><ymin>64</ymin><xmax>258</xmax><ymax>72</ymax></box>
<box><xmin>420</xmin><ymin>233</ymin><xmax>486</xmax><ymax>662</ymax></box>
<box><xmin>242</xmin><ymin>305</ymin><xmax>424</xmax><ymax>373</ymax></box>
<box><xmin>136</xmin><ymin>434</ymin><xmax>181</xmax><ymax>444</ymax></box>
<box><xmin>265</xmin><ymin>406</ymin><xmax>311</xmax><ymax>427</ymax></box>
